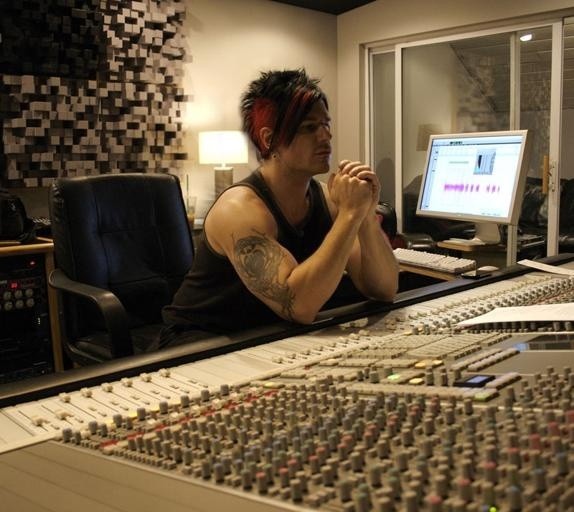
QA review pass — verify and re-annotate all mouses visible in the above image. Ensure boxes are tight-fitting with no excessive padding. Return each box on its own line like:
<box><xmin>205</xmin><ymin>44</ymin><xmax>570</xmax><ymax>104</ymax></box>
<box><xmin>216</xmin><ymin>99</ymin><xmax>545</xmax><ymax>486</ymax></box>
<box><xmin>478</xmin><ymin>265</ymin><xmax>499</xmax><ymax>271</ymax></box>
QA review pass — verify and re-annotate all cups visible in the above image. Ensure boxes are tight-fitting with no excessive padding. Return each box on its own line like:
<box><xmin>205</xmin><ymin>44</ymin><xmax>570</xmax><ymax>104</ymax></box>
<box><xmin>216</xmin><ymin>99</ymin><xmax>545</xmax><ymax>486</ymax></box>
<box><xmin>184</xmin><ymin>196</ymin><xmax>196</xmax><ymax>226</ymax></box>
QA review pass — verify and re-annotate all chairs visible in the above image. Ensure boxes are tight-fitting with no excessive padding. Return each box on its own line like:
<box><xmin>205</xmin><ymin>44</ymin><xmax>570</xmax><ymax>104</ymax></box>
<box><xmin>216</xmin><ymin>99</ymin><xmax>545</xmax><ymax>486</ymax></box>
<box><xmin>48</xmin><ymin>171</ymin><xmax>195</xmax><ymax>366</ymax></box>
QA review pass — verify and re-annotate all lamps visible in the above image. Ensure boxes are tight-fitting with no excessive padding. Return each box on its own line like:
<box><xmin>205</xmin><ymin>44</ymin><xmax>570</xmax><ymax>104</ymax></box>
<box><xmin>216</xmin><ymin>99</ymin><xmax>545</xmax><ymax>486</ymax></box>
<box><xmin>199</xmin><ymin>130</ymin><xmax>249</xmax><ymax>202</ymax></box>
<box><xmin>417</xmin><ymin>123</ymin><xmax>444</xmax><ymax>151</ymax></box>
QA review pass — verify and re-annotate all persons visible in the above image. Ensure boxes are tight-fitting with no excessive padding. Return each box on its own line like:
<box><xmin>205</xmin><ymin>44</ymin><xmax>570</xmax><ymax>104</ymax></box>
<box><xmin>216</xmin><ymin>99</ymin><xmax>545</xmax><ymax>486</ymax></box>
<box><xmin>147</xmin><ymin>68</ymin><xmax>399</xmax><ymax>356</ymax></box>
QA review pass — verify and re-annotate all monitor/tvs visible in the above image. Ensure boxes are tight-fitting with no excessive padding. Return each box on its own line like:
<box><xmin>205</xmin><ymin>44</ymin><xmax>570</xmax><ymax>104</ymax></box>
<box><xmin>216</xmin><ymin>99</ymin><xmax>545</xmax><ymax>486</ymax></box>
<box><xmin>415</xmin><ymin>129</ymin><xmax>535</xmax><ymax>251</ymax></box>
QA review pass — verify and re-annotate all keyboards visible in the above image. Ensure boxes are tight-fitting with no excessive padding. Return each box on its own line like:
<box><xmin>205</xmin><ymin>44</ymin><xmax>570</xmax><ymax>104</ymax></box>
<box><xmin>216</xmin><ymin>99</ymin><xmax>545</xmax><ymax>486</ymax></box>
<box><xmin>393</xmin><ymin>248</ymin><xmax>477</xmax><ymax>275</ymax></box>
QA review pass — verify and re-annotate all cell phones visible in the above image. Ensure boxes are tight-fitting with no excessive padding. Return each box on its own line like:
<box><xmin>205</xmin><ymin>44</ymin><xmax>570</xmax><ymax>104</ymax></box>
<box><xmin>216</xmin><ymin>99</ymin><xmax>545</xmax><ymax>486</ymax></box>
<box><xmin>460</xmin><ymin>269</ymin><xmax>492</xmax><ymax>278</ymax></box>
